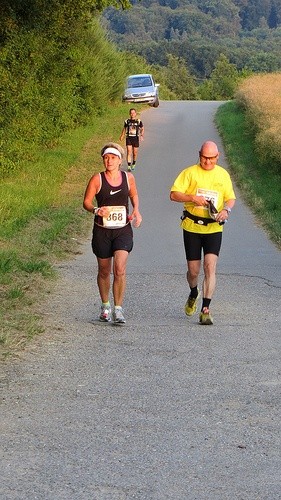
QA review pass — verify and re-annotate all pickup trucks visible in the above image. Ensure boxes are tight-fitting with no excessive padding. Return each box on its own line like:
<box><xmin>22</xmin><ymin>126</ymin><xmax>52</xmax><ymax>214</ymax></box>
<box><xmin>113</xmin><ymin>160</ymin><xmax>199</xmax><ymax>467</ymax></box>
<box><xmin>120</xmin><ymin>73</ymin><xmax>159</xmax><ymax>107</ymax></box>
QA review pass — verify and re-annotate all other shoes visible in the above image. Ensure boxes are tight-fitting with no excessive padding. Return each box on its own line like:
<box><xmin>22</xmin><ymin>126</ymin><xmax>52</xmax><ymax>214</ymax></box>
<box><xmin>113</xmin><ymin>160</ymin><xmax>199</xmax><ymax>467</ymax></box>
<box><xmin>127</xmin><ymin>166</ymin><xmax>131</xmax><ymax>171</ymax></box>
<box><xmin>131</xmin><ymin>163</ymin><xmax>135</xmax><ymax>170</ymax></box>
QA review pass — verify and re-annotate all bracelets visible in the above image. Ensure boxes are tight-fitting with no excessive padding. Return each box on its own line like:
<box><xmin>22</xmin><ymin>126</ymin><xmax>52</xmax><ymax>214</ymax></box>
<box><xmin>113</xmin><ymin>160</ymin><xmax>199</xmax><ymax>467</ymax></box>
<box><xmin>141</xmin><ymin>135</ymin><xmax>143</xmax><ymax>136</ymax></box>
<box><xmin>89</xmin><ymin>207</ymin><xmax>100</xmax><ymax>215</ymax></box>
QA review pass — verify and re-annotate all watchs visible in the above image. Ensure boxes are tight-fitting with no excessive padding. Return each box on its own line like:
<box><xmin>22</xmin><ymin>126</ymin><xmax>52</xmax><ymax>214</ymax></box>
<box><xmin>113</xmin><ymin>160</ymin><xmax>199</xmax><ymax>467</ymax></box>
<box><xmin>222</xmin><ymin>208</ymin><xmax>231</xmax><ymax>214</ymax></box>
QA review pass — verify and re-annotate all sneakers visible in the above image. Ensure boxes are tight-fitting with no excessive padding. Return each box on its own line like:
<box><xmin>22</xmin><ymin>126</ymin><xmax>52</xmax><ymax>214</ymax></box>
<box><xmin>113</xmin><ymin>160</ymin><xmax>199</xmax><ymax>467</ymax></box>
<box><xmin>98</xmin><ymin>305</ymin><xmax>111</xmax><ymax>322</ymax></box>
<box><xmin>185</xmin><ymin>287</ymin><xmax>200</xmax><ymax>316</ymax></box>
<box><xmin>198</xmin><ymin>307</ymin><xmax>213</xmax><ymax>325</ymax></box>
<box><xmin>111</xmin><ymin>308</ymin><xmax>125</xmax><ymax>323</ymax></box>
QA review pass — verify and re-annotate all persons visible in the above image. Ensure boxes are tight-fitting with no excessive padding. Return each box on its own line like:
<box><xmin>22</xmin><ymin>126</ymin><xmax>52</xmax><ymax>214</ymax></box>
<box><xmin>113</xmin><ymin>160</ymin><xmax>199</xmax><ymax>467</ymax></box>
<box><xmin>120</xmin><ymin>108</ymin><xmax>144</xmax><ymax>172</ymax></box>
<box><xmin>170</xmin><ymin>142</ymin><xmax>236</xmax><ymax>325</ymax></box>
<box><xmin>83</xmin><ymin>142</ymin><xmax>142</xmax><ymax>323</ymax></box>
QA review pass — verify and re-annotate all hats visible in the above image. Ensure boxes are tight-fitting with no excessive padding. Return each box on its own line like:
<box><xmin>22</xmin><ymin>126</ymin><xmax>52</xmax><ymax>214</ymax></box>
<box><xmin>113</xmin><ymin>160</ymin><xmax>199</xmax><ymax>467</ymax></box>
<box><xmin>102</xmin><ymin>147</ymin><xmax>121</xmax><ymax>159</ymax></box>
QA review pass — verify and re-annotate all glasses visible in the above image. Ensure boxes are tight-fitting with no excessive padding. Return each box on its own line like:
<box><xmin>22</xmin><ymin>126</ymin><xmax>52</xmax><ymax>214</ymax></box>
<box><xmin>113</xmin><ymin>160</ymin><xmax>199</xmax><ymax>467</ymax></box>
<box><xmin>200</xmin><ymin>154</ymin><xmax>217</xmax><ymax>160</ymax></box>
